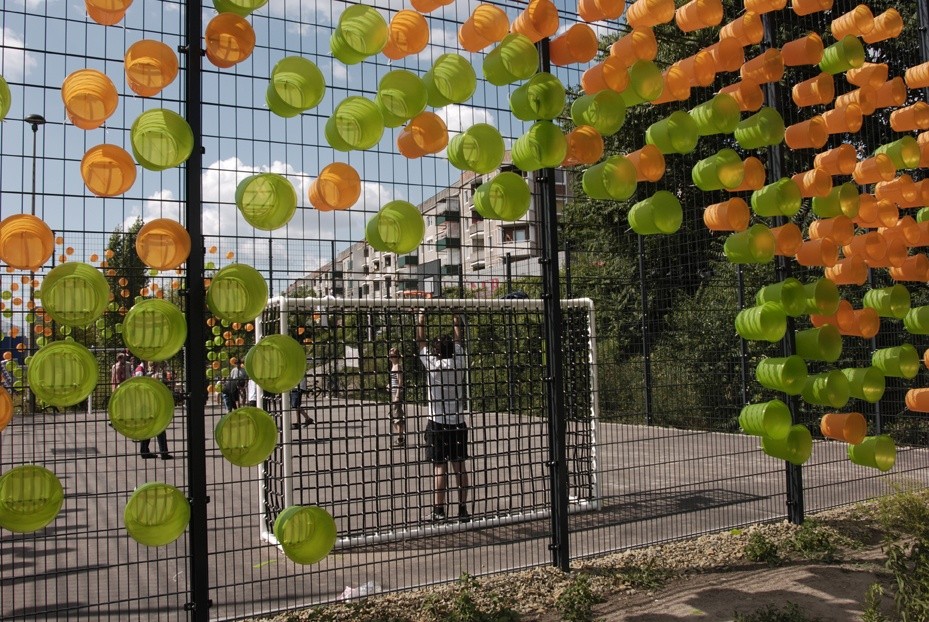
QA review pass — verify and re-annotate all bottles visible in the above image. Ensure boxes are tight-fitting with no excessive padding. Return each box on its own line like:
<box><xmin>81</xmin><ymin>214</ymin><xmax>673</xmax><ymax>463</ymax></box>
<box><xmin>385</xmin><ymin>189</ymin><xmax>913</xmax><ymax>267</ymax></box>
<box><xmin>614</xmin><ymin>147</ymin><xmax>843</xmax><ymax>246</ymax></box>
<box><xmin>0</xmin><ymin>214</ymin><xmax>55</xmax><ymax>271</ymax></box>
<box><xmin>0</xmin><ymin>75</ymin><xmax>12</xmax><ymax>120</ymax></box>
<box><xmin>0</xmin><ymin>387</ymin><xmax>13</xmax><ymax>431</ymax></box>
<box><xmin>80</xmin><ymin>145</ymin><xmax>138</xmax><ymax>197</ymax></box>
<box><xmin>61</xmin><ymin>68</ymin><xmax>119</xmax><ymax>130</ymax></box>
<box><xmin>39</xmin><ymin>260</ymin><xmax>109</xmax><ymax>326</ymax></box>
<box><xmin>29</xmin><ymin>342</ymin><xmax>99</xmax><ymax>407</ymax></box>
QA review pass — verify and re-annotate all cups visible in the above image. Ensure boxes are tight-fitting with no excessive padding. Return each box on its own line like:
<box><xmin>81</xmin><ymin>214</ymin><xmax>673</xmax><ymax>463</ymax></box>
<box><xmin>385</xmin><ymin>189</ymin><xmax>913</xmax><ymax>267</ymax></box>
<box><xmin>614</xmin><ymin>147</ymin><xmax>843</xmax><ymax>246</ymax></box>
<box><xmin>89</xmin><ymin>1</ymin><xmax>928</xmax><ymax>469</ymax></box>
<box><xmin>272</xmin><ymin>504</ymin><xmax>338</xmax><ymax>566</ymax></box>
<box><xmin>122</xmin><ymin>482</ymin><xmax>189</xmax><ymax>545</ymax></box>
<box><xmin>0</xmin><ymin>464</ymin><xmax>65</xmax><ymax>534</ymax></box>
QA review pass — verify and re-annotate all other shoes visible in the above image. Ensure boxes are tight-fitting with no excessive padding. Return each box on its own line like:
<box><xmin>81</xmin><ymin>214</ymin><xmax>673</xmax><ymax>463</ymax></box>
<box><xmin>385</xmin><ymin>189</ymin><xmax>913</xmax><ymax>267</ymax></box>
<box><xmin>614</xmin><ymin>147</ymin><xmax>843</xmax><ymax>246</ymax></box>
<box><xmin>162</xmin><ymin>454</ymin><xmax>174</xmax><ymax>460</ymax></box>
<box><xmin>394</xmin><ymin>437</ymin><xmax>405</xmax><ymax>447</ymax></box>
<box><xmin>291</xmin><ymin>423</ymin><xmax>299</xmax><ymax>429</ymax></box>
<box><xmin>304</xmin><ymin>418</ymin><xmax>315</xmax><ymax>429</ymax></box>
<box><xmin>457</xmin><ymin>514</ymin><xmax>470</xmax><ymax>523</ymax></box>
<box><xmin>420</xmin><ymin>509</ymin><xmax>447</xmax><ymax>522</ymax></box>
<box><xmin>142</xmin><ymin>452</ymin><xmax>158</xmax><ymax>458</ymax></box>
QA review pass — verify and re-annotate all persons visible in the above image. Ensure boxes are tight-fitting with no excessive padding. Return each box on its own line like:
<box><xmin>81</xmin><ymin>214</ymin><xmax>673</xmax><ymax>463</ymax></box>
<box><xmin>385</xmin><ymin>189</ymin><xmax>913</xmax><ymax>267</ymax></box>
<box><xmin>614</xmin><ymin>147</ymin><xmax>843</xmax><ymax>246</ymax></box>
<box><xmin>215</xmin><ymin>358</ymin><xmax>257</xmax><ymax>413</ymax></box>
<box><xmin>417</xmin><ymin>305</ymin><xmax>472</xmax><ymax>523</ymax></box>
<box><xmin>387</xmin><ymin>348</ymin><xmax>405</xmax><ymax>447</ymax></box>
<box><xmin>290</xmin><ymin>376</ymin><xmax>314</xmax><ymax>430</ymax></box>
<box><xmin>108</xmin><ymin>353</ymin><xmax>174</xmax><ymax>460</ymax></box>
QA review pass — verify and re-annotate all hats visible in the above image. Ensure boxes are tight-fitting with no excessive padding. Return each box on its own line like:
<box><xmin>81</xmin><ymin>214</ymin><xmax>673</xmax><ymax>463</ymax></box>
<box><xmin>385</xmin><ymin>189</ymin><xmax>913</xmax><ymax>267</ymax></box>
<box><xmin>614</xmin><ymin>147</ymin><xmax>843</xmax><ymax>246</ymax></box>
<box><xmin>433</xmin><ymin>335</ymin><xmax>454</xmax><ymax>355</ymax></box>
